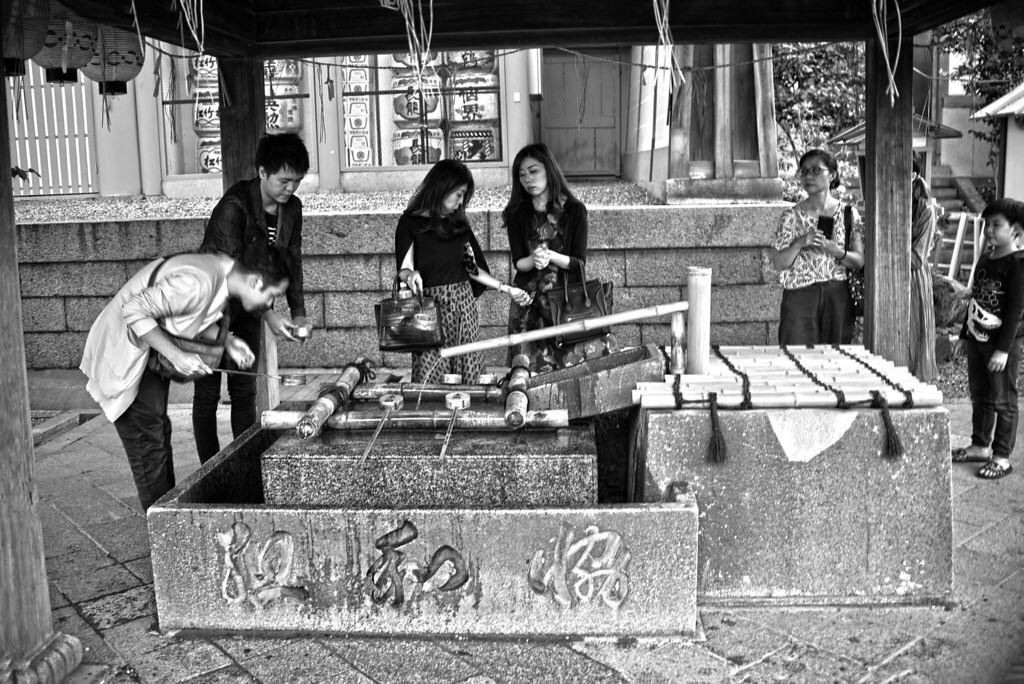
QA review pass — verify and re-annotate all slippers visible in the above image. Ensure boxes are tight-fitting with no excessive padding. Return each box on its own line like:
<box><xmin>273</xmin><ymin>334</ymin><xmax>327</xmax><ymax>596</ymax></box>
<box><xmin>952</xmin><ymin>448</ymin><xmax>991</xmax><ymax>461</ymax></box>
<box><xmin>979</xmin><ymin>461</ymin><xmax>1012</xmax><ymax>478</ymax></box>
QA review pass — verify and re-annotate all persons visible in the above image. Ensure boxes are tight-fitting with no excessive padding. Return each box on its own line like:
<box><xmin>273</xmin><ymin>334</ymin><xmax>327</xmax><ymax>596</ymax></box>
<box><xmin>910</xmin><ymin>150</ymin><xmax>941</xmax><ymax>386</ymax></box>
<box><xmin>501</xmin><ymin>143</ymin><xmax>615</xmax><ymax>368</ymax></box>
<box><xmin>394</xmin><ymin>141</ymin><xmax>533</xmax><ymax>385</ymax></box>
<box><xmin>192</xmin><ymin>132</ymin><xmax>314</xmax><ymax>465</ymax></box>
<box><xmin>951</xmin><ymin>197</ymin><xmax>1024</xmax><ymax>479</ymax></box>
<box><xmin>771</xmin><ymin>149</ymin><xmax>865</xmax><ymax>346</ymax></box>
<box><xmin>78</xmin><ymin>242</ymin><xmax>298</xmax><ymax>515</ymax></box>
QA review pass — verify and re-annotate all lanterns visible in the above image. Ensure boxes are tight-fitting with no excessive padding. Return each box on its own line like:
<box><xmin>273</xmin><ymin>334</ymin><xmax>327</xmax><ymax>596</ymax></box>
<box><xmin>0</xmin><ymin>0</ymin><xmax>145</xmax><ymax>95</ymax></box>
<box><xmin>187</xmin><ymin>51</ymin><xmax>304</xmax><ymax>174</ymax></box>
<box><xmin>392</xmin><ymin>48</ymin><xmax>500</xmax><ymax>165</ymax></box>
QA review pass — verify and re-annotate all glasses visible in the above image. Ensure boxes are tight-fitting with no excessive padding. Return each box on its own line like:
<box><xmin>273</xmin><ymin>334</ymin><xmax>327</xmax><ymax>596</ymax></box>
<box><xmin>800</xmin><ymin>167</ymin><xmax>829</xmax><ymax>176</ymax></box>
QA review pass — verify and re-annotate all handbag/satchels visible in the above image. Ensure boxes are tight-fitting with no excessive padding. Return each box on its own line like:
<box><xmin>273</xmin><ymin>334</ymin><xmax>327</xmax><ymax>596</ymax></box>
<box><xmin>148</xmin><ymin>328</ymin><xmax>226</xmax><ymax>384</ymax></box>
<box><xmin>844</xmin><ymin>205</ymin><xmax>864</xmax><ymax>305</ymax></box>
<box><xmin>374</xmin><ymin>268</ymin><xmax>445</xmax><ymax>352</ymax></box>
<box><xmin>538</xmin><ymin>259</ymin><xmax>613</xmax><ymax>351</ymax></box>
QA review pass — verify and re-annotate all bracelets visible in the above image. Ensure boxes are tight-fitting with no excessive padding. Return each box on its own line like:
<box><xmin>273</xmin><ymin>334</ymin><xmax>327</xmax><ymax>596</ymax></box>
<box><xmin>835</xmin><ymin>248</ymin><xmax>847</xmax><ymax>261</ymax></box>
<box><xmin>497</xmin><ymin>281</ymin><xmax>505</xmax><ymax>293</ymax></box>
<box><xmin>405</xmin><ymin>270</ymin><xmax>421</xmax><ymax>283</ymax></box>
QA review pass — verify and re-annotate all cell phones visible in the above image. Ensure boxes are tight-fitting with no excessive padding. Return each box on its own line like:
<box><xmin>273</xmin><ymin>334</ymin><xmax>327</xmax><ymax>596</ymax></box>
<box><xmin>817</xmin><ymin>215</ymin><xmax>835</xmax><ymax>240</ymax></box>
